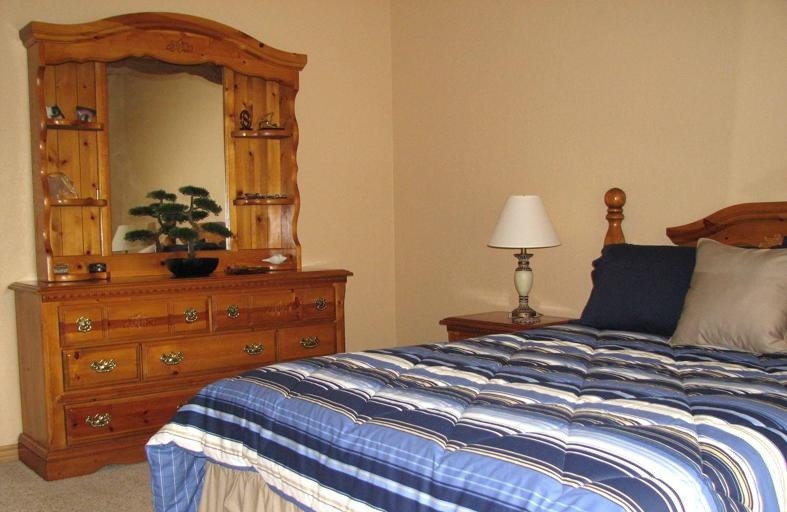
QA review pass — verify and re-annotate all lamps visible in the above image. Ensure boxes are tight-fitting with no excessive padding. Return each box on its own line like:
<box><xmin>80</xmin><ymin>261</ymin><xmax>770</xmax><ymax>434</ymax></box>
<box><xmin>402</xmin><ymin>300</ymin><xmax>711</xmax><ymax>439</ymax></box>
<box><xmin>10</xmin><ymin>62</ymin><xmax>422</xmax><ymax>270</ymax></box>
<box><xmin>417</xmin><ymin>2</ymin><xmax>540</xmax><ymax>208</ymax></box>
<box><xmin>487</xmin><ymin>192</ymin><xmax>565</xmax><ymax>332</ymax></box>
<box><xmin>111</xmin><ymin>224</ymin><xmax>144</xmax><ymax>255</ymax></box>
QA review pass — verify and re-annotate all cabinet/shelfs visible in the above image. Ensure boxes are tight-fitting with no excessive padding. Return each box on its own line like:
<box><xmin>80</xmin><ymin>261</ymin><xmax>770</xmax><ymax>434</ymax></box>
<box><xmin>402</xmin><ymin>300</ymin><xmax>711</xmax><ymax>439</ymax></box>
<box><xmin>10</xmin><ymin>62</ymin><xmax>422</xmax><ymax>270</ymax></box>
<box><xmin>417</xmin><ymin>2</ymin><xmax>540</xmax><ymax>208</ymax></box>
<box><xmin>4</xmin><ymin>265</ymin><xmax>355</xmax><ymax>482</ymax></box>
<box><xmin>35</xmin><ymin>45</ymin><xmax>109</xmax><ymax>280</ymax></box>
<box><xmin>230</xmin><ymin>55</ymin><xmax>301</xmax><ymax>269</ymax></box>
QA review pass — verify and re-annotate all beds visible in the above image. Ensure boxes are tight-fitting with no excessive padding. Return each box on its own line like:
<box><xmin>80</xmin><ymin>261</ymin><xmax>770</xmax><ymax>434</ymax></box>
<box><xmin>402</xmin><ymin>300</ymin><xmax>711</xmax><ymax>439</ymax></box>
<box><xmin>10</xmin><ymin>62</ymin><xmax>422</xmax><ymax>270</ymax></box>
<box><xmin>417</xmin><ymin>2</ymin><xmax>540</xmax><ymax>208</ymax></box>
<box><xmin>144</xmin><ymin>185</ymin><xmax>786</xmax><ymax>509</ymax></box>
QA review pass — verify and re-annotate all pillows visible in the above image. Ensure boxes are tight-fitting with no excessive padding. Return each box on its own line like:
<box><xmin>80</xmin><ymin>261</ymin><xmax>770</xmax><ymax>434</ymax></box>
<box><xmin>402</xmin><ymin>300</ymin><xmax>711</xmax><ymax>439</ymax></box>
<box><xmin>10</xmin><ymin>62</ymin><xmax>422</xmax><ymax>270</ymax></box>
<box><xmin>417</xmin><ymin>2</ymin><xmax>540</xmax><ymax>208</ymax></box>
<box><xmin>580</xmin><ymin>239</ymin><xmax>697</xmax><ymax>341</ymax></box>
<box><xmin>666</xmin><ymin>237</ymin><xmax>786</xmax><ymax>353</ymax></box>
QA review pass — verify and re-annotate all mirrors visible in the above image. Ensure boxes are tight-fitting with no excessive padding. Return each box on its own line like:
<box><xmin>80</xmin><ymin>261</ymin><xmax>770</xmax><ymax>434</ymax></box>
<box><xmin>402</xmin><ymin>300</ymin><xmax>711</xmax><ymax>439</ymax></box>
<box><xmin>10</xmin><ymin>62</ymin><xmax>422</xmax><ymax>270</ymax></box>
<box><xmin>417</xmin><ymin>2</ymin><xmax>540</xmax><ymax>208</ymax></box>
<box><xmin>98</xmin><ymin>52</ymin><xmax>238</xmax><ymax>260</ymax></box>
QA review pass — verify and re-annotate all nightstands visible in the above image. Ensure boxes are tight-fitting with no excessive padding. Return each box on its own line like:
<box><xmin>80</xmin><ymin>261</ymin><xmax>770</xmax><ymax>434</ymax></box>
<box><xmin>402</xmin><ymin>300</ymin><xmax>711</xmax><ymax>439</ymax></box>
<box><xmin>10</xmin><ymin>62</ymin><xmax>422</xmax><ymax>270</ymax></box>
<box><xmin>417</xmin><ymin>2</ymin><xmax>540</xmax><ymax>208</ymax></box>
<box><xmin>439</xmin><ymin>308</ymin><xmax>579</xmax><ymax>349</ymax></box>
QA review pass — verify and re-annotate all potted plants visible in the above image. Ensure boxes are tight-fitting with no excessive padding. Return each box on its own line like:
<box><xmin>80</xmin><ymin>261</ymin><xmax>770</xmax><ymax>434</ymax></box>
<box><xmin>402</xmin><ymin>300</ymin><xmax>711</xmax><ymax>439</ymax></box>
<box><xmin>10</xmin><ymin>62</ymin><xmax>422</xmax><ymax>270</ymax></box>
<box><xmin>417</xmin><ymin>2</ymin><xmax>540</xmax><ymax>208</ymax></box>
<box><xmin>163</xmin><ymin>182</ymin><xmax>228</xmax><ymax>283</ymax></box>
<box><xmin>123</xmin><ymin>184</ymin><xmax>184</xmax><ymax>257</ymax></box>
